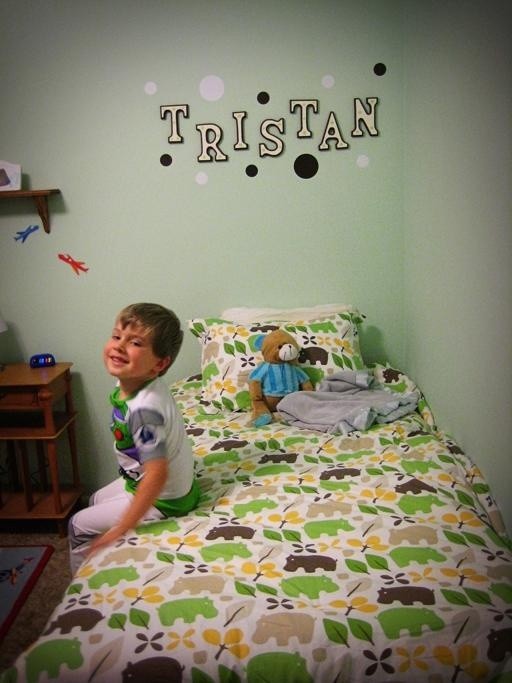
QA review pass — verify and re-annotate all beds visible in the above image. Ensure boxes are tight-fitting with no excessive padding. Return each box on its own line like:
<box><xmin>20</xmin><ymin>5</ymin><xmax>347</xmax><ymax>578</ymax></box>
<box><xmin>1</xmin><ymin>366</ymin><xmax>512</xmax><ymax>682</ymax></box>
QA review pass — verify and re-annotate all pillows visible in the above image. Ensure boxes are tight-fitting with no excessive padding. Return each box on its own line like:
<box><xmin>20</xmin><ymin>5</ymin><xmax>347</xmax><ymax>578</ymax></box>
<box><xmin>187</xmin><ymin>304</ymin><xmax>369</xmax><ymax>415</ymax></box>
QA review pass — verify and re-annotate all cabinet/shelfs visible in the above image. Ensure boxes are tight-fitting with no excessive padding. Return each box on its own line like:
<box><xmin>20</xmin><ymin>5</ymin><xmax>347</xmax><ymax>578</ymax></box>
<box><xmin>0</xmin><ymin>361</ymin><xmax>86</xmax><ymax>539</ymax></box>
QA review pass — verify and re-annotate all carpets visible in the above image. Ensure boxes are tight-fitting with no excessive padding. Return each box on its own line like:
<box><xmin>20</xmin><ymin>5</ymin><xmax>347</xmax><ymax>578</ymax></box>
<box><xmin>1</xmin><ymin>543</ymin><xmax>55</xmax><ymax>643</ymax></box>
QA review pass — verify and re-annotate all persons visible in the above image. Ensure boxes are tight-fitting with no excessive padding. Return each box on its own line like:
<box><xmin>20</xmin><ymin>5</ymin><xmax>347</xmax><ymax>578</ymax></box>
<box><xmin>64</xmin><ymin>300</ymin><xmax>201</xmax><ymax>576</ymax></box>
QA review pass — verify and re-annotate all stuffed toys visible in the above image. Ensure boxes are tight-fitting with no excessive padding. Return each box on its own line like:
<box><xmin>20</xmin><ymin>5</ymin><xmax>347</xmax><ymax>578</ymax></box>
<box><xmin>247</xmin><ymin>328</ymin><xmax>315</xmax><ymax>427</ymax></box>
